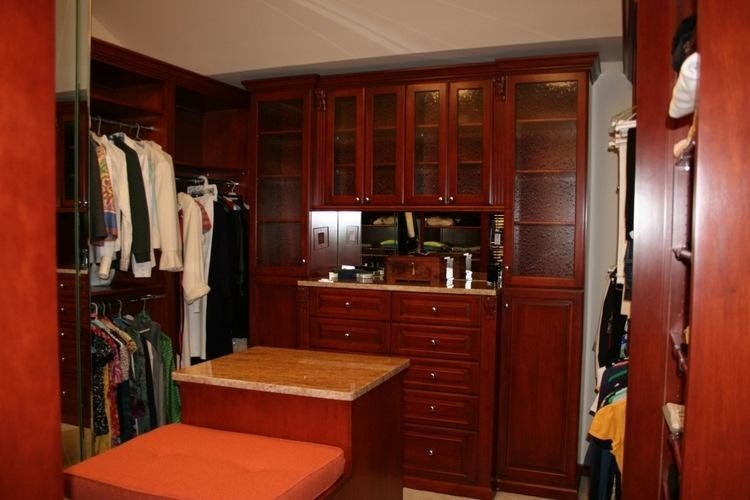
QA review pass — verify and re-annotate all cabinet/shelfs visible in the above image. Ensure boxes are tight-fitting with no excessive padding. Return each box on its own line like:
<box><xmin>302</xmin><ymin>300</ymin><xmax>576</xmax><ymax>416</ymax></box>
<box><xmin>247</xmin><ymin>272</ymin><xmax>310</xmax><ymax>350</ymax></box>
<box><xmin>602</xmin><ymin>1</ymin><xmax>749</xmax><ymax>500</ymax></box>
<box><xmin>295</xmin><ymin>279</ymin><xmax>501</xmax><ymax>498</ymax></box>
<box><xmin>399</xmin><ymin>77</ymin><xmax>494</xmax><ymax>211</ymax></box>
<box><xmin>490</xmin><ymin>278</ymin><xmax>578</xmax><ymax>495</ymax></box>
<box><xmin>62</xmin><ymin>343</ymin><xmax>413</xmax><ymax>500</ymax></box>
<box><xmin>55</xmin><ymin>37</ymin><xmax>246</xmax><ymax>431</ymax></box>
<box><xmin>246</xmin><ymin>86</ymin><xmax>315</xmax><ymax>276</ymax></box>
<box><xmin>312</xmin><ymin>82</ymin><xmax>404</xmax><ymax>209</ymax></box>
<box><xmin>501</xmin><ymin>70</ymin><xmax>587</xmax><ymax>292</ymax></box>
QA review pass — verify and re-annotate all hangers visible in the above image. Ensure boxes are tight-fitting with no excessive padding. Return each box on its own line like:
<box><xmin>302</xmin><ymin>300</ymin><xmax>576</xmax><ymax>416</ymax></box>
<box><xmin>174</xmin><ymin>172</ymin><xmax>254</xmax><ymax>215</ymax></box>
<box><xmin>90</xmin><ymin>294</ymin><xmax>158</xmax><ymax>331</ymax></box>
<box><xmin>94</xmin><ymin>116</ymin><xmax>145</xmax><ymax>143</ymax></box>
